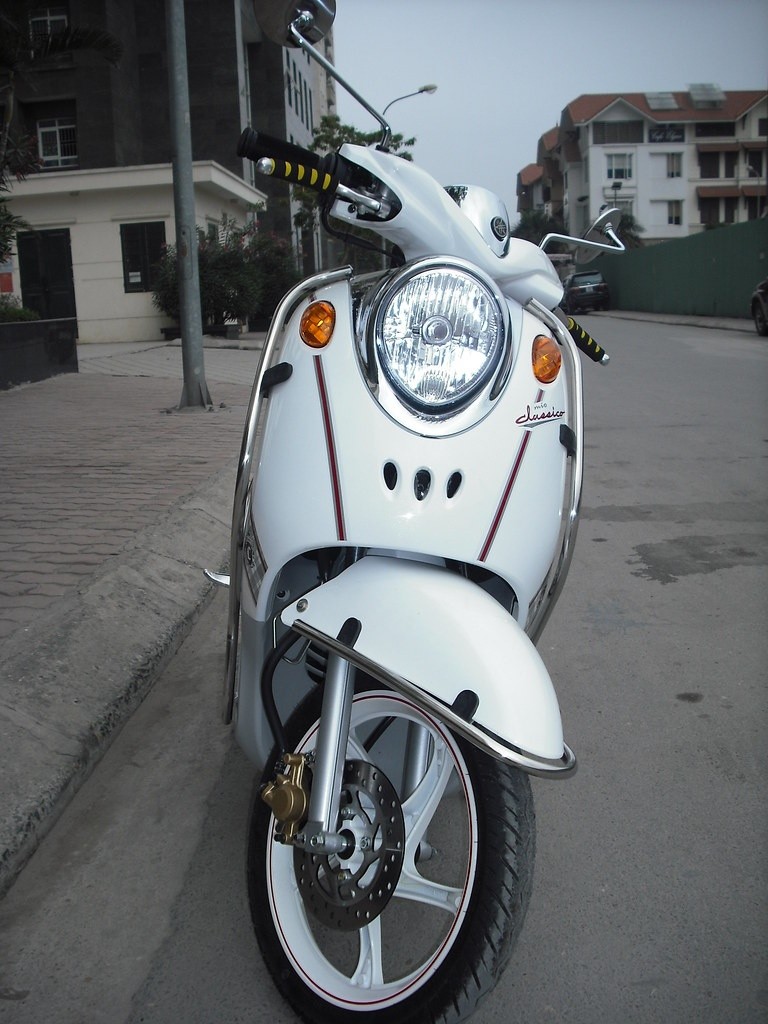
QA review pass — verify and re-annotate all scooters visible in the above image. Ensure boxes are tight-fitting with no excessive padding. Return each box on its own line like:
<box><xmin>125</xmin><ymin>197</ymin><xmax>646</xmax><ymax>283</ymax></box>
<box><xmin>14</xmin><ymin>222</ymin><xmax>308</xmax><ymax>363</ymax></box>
<box><xmin>227</xmin><ymin>1</ymin><xmax>629</xmax><ymax>1023</ymax></box>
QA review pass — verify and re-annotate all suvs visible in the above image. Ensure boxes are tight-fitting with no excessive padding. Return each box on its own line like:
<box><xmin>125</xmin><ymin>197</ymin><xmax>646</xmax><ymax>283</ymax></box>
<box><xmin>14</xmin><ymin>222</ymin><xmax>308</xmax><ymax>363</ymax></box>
<box><xmin>561</xmin><ymin>270</ymin><xmax>611</xmax><ymax>313</ymax></box>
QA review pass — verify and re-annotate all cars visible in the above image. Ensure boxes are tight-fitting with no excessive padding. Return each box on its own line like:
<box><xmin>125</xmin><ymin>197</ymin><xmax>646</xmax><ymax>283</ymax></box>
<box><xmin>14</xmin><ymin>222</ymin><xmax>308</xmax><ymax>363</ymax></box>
<box><xmin>751</xmin><ymin>277</ymin><xmax>768</xmax><ymax>335</ymax></box>
